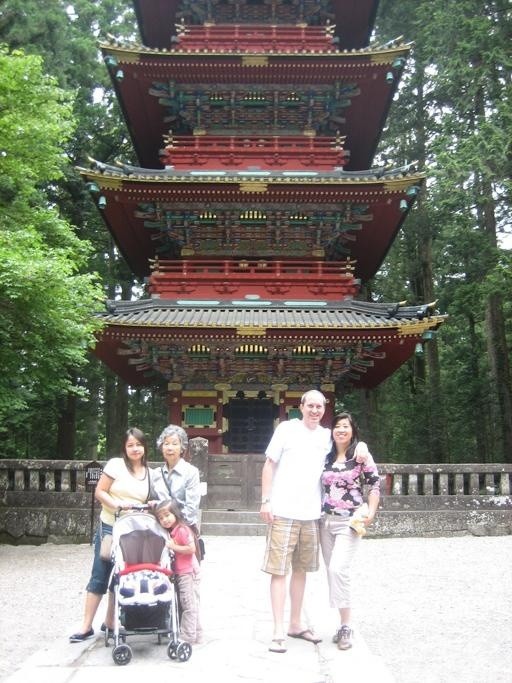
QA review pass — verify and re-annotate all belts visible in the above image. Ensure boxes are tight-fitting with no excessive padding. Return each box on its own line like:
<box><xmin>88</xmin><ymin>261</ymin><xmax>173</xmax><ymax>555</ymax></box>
<box><xmin>325</xmin><ymin>509</ymin><xmax>353</xmax><ymax>517</ymax></box>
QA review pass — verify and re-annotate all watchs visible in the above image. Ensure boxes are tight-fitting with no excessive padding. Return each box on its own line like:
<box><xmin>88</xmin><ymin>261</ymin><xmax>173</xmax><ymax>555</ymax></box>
<box><xmin>260</xmin><ymin>496</ymin><xmax>270</xmax><ymax>504</ymax></box>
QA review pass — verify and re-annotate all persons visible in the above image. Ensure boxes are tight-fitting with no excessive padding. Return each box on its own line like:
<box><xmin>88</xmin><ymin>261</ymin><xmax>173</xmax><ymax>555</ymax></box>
<box><xmin>319</xmin><ymin>411</ymin><xmax>381</xmax><ymax>650</ymax></box>
<box><xmin>259</xmin><ymin>389</ymin><xmax>369</xmax><ymax>652</ymax></box>
<box><xmin>156</xmin><ymin>499</ymin><xmax>201</xmax><ymax>646</ymax></box>
<box><xmin>69</xmin><ymin>427</ymin><xmax>161</xmax><ymax>642</ymax></box>
<box><xmin>153</xmin><ymin>424</ymin><xmax>201</xmax><ymax>623</ymax></box>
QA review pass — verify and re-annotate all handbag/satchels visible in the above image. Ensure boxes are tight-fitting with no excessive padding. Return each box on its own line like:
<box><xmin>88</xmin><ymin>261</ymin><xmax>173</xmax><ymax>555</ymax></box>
<box><xmin>198</xmin><ymin>539</ymin><xmax>205</xmax><ymax>560</ymax></box>
<box><xmin>99</xmin><ymin>533</ymin><xmax>113</xmax><ymax>563</ymax></box>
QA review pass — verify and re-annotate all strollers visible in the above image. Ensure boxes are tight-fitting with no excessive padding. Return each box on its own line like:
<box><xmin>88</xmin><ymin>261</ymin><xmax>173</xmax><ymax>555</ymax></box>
<box><xmin>105</xmin><ymin>499</ymin><xmax>196</xmax><ymax>666</ymax></box>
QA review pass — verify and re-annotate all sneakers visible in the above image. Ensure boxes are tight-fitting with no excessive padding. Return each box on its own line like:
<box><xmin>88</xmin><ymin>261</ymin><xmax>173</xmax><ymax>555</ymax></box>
<box><xmin>69</xmin><ymin>625</ymin><xmax>94</xmax><ymax>642</ymax></box>
<box><xmin>332</xmin><ymin>624</ymin><xmax>355</xmax><ymax>650</ymax></box>
<box><xmin>100</xmin><ymin>622</ymin><xmax>114</xmax><ymax>633</ymax></box>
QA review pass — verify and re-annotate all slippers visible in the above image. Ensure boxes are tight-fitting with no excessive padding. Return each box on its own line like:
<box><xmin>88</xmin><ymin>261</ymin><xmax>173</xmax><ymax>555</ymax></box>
<box><xmin>288</xmin><ymin>625</ymin><xmax>322</xmax><ymax>642</ymax></box>
<box><xmin>269</xmin><ymin>638</ymin><xmax>287</xmax><ymax>653</ymax></box>
